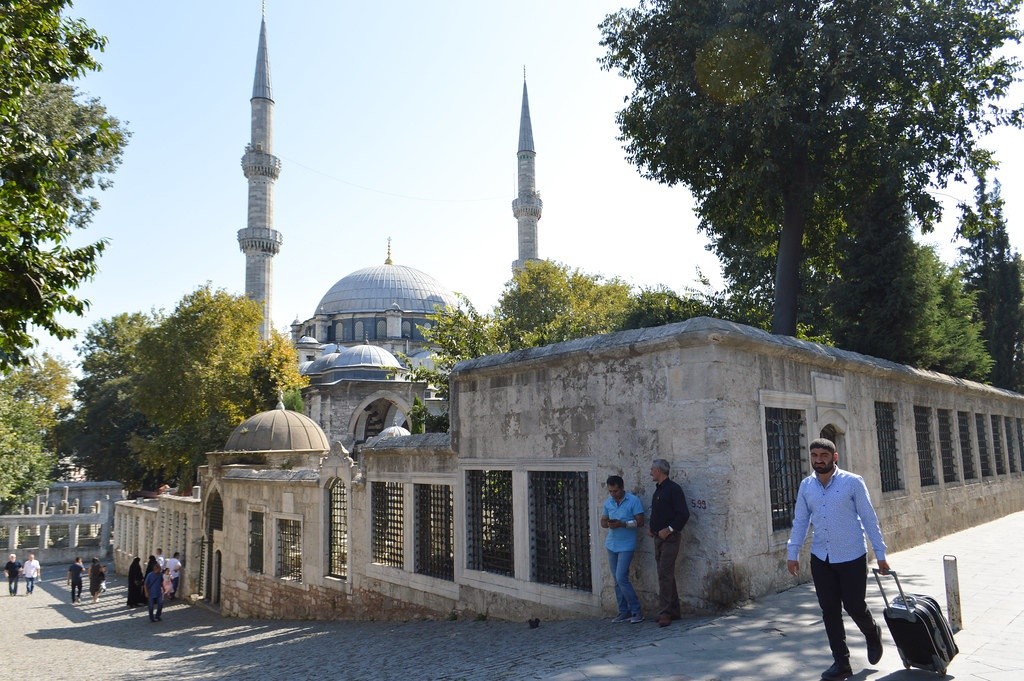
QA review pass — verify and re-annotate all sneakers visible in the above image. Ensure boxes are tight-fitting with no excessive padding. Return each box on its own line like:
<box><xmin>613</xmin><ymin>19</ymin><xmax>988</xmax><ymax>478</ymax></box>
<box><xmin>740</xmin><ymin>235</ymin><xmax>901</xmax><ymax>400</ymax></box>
<box><xmin>821</xmin><ymin>661</ymin><xmax>853</xmax><ymax>680</ymax></box>
<box><xmin>867</xmin><ymin>625</ymin><xmax>883</xmax><ymax>665</ymax></box>
<box><xmin>611</xmin><ymin>612</ymin><xmax>645</xmax><ymax>623</ymax></box>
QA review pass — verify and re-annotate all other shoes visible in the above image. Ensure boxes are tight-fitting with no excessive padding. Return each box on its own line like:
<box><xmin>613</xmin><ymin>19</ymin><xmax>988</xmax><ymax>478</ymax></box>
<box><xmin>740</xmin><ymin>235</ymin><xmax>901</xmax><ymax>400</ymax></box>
<box><xmin>72</xmin><ymin>598</ymin><xmax>81</xmax><ymax>603</ymax></box>
<box><xmin>11</xmin><ymin>593</ymin><xmax>16</xmax><ymax>596</ymax></box>
<box><xmin>151</xmin><ymin>619</ymin><xmax>161</xmax><ymax>622</ymax></box>
<box><xmin>26</xmin><ymin>592</ymin><xmax>33</xmax><ymax>595</ymax></box>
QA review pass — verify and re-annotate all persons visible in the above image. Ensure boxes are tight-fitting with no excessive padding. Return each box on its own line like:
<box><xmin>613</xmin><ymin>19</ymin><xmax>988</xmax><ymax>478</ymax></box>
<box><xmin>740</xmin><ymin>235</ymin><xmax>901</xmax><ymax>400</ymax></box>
<box><xmin>786</xmin><ymin>438</ymin><xmax>890</xmax><ymax>681</ymax></box>
<box><xmin>23</xmin><ymin>554</ymin><xmax>40</xmax><ymax>594</ymax></box>
<box><xmin>600</xmin><ymin>475</ymin><xmax>645</xmax><ymax>623</ymax></box>
<box><xmin>89</xmin><ymin>557</ymin><xmax>106</xmax><ymax>604</ymax></box>
<box><xmin>649</xmin><ymin>458</ymin><xmax>690</xmax><ymax>626</ymax></box>
<box><xmin>4</xmin><ymin>554</ymin><xmax>23</xmax><ymax>596</ymax></box>
<box><xmin>67</xmin><ymin>557</ymin><xmax>85</xmax><ymax>604</ymax></box>
<box><xmin>127</xmin><ymin>548</ymin><xmax>182</xmax><ymax>622</ymax></box>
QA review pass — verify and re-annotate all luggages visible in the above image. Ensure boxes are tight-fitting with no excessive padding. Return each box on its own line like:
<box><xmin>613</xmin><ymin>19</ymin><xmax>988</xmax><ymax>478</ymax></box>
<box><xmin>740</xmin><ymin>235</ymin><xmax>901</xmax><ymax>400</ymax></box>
<box><xmin>872</xmin><ymin>569</ymin><xmax>959</xmax><ymax>679</ymax></box>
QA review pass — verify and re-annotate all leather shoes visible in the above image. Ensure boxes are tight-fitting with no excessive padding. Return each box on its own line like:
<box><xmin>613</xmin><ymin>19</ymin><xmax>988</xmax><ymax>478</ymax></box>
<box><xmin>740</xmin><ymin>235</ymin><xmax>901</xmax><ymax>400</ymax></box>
<box><xmin>654</xmin><ymin>614</ymin><xmax>672</xmax><ymax>627</ymax></box>
